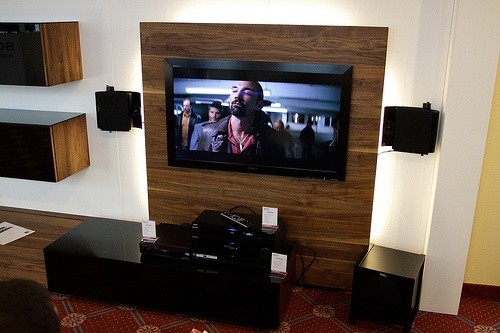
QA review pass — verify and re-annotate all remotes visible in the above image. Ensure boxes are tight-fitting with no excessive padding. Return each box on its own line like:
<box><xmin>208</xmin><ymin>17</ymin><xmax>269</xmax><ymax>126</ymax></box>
<box><xmin>220</xmin><ymin>211</ymin><xmax>255</xmax><ymax>228</ymax></box>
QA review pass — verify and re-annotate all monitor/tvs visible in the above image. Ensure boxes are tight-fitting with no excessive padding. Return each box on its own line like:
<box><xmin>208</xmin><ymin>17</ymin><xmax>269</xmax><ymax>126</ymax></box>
<box><xmin>164</xmin><ymin>58</ymin><xmax>353</xmax><ymax>183</ymax></box>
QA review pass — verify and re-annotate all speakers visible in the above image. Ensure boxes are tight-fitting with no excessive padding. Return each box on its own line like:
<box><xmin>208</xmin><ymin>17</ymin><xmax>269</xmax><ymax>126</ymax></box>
<box><xmin>393</xmin><ymin>107</ymin><xmax>439</xmax><ymax>156</ymax></box>
<box><xmin>95</xmin><ymin>91</ymin><xmax>132</xmax><ymax>132</ymax></box>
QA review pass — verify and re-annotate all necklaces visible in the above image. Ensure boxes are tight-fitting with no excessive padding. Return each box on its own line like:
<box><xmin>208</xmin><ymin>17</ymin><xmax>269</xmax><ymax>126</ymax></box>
<box><xmin>231</xmin><ymin>123</ymin><xmax>259</xmax><ymax>152</ymax></box>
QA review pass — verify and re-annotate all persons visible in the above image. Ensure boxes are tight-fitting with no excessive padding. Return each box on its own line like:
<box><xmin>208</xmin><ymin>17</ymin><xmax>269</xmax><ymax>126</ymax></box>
<box><xmin>190</xmin><ymin>103</ymin><xmax>222</xmax><ymax>150</ymax></box>
<box><xmin>190</xmin><ymin>80</ymin><xmax>284</xmax><ymax>158</ymax></box>
<box><xmin>174</xmin><ymin>98</ymin><xmax>201</xmax><ymax>151</ymax></box>
<box><xmin>274</xmin><ymin>119</ymin><xmax>296</xmax><ymax>158</ymax></box>
<box><xmin>299</xmin><ymin>119</ymin><xmax>316</xmax><ymax>159</ymax></box>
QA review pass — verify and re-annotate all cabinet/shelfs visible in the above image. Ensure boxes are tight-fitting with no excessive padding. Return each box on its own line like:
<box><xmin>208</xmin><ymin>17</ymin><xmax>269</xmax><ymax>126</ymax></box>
<box><xmin>0</xmin><ymin>22</ymin><xmax>83</xmax><ymax>86</ymax></box>
<box><xmin>42</xmin><ymin>218</ymin><xmax>299</xmax><ymax>329</ymax></box>
<box><xmin>0</xmin><ymin>108</ymin><xmax>91</xmax><ymax>183</ymax></box>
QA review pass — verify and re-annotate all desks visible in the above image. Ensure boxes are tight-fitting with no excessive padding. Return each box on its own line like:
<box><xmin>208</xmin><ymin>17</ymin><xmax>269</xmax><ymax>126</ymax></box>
<box><xmin>349</xmin><ymin>244</ymin><xmax>425</xmax><ymax>332</ymax></box>
